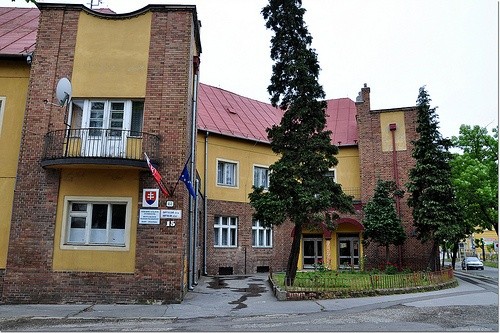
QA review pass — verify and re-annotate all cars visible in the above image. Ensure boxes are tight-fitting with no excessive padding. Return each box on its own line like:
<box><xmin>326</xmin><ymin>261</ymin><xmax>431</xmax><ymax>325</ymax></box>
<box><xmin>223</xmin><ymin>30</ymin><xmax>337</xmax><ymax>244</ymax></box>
<box><xmin>460</xmin><ymin>256</ymin><xmax>484</xmax><ymax>270</ymax></box>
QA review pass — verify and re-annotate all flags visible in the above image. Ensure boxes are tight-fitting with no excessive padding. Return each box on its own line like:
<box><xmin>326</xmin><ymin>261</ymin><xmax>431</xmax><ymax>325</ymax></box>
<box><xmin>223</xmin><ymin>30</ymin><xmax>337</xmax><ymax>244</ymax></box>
<box><xmin>179</xmin><ymin>166</ymin><xmax>197</xmax><ymax>199</ymax></box>
<box><xmin>144</xmin><ymin>153</ymin><xmax>169</xmax><ymax>197</ymax></box>
